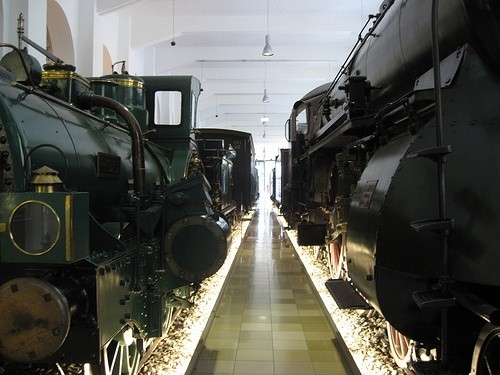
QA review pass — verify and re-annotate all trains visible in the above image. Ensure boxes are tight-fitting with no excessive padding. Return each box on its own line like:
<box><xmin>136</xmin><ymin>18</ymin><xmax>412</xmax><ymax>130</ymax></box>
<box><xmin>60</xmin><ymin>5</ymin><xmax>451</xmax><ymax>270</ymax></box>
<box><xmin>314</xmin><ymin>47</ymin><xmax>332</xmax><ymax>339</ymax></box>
<box><xmin>0</xmin><ymin>11</ymin><xmax>258</xmax><ymax>368</ymax></box>
<box><xmin>271</xmin><ymin>0</ymin><xmax>499</xmax><ymax>375</ymax></box>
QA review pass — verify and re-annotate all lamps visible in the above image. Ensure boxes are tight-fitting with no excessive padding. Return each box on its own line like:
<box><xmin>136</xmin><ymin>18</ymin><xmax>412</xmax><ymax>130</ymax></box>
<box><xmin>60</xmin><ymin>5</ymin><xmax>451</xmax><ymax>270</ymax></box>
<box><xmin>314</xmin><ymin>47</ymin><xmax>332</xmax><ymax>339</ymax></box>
<box><xmin>262</xmin><ymin>61</ymin><xmax>270</xmax><ymax>103</ymax></box>
<box><xmin>262</xmin><ymin>0</ymin><xmax>274</xmax><ymax>57</ymax></box>
